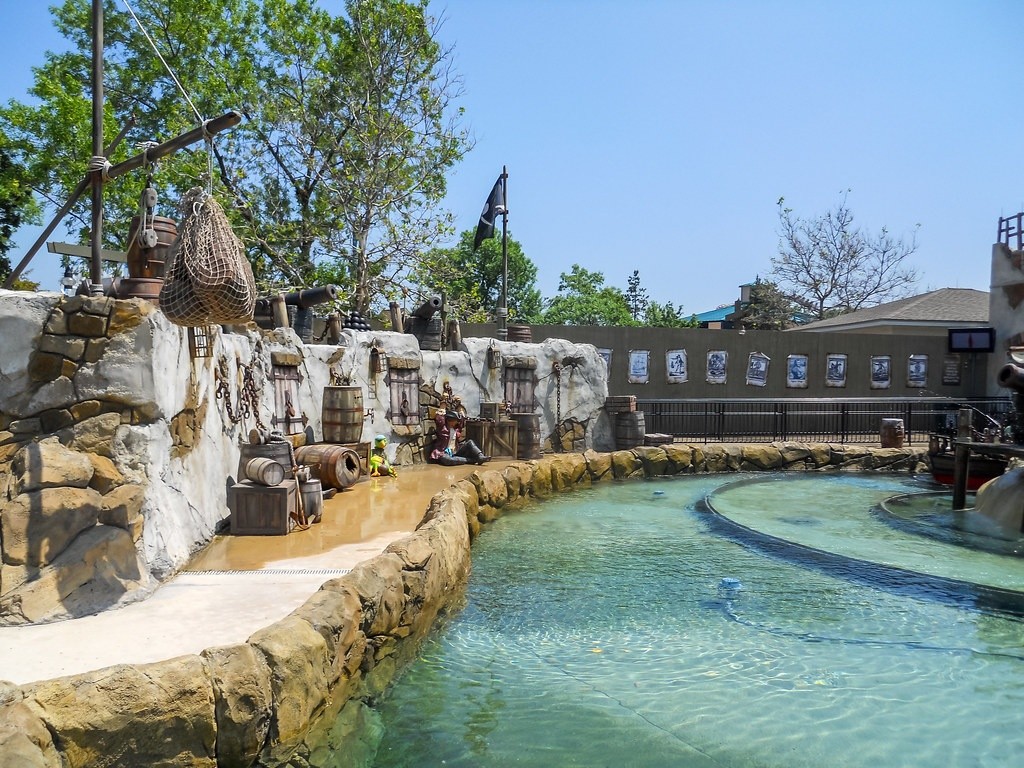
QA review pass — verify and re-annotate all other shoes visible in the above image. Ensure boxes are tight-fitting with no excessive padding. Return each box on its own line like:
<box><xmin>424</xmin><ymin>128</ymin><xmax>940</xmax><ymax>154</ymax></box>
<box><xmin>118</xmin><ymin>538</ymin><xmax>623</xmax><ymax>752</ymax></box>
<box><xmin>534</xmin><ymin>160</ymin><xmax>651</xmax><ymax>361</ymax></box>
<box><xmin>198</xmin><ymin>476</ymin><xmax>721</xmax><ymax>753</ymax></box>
<box><xmin>469</xmin><ymin>455</ymin><xmax>491</xmax><ymax>466</ymax></box>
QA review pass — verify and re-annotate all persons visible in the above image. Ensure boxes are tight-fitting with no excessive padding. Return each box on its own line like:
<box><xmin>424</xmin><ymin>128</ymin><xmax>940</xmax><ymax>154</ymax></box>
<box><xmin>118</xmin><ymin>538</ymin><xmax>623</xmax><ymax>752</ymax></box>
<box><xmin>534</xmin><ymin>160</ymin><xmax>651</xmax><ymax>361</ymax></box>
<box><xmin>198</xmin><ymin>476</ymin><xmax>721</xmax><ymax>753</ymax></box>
<box><xmin>428</xmin><ymin>392</ymin><xmax>491</xmax><ymax>467</ymax></box>
<box><xmin>367</xmin><ymin>435</ymin><xmax>398</xmax><ymax>478</ymax></box>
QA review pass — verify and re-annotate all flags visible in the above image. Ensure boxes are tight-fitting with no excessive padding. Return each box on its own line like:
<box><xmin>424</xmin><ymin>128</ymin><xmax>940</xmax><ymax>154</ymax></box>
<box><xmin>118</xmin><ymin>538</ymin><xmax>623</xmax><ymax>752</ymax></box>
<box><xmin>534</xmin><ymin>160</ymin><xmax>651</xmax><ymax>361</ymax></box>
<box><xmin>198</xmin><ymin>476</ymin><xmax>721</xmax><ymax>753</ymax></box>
<box><xmin>472</xmin><ymin>175</ymin><xmax>506</xmax><ymax>254</ymax></box>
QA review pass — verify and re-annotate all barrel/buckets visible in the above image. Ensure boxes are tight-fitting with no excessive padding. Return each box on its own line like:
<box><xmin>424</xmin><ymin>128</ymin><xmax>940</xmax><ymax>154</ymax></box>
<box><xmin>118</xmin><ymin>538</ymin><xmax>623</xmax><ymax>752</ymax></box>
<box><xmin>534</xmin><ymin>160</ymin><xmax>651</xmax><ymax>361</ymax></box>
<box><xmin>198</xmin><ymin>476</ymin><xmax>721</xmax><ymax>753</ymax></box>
<box><xmin>293</xmin><ymin>445</ymin><xmax>361</xmax><ymax>490</ymax></box>
<box><xmin>880</xmin><ymin>418</ymin><xmax>905</xmax><ymax>448</ymax></box>
<box><xmin>322</xmin><ymin>386</ymin><xmax>364</xmax><ymax>443</ymax></box>
<box><xmin>244</xmin><ymin>457</ymin><xmax>285</xmax><ymax>486</ymax></box>
<box><xmin>507</xmin><ymin>325</ymin><xmax>532</xmax><ymax>344</ymax></box>
<box><xmin>615</xmin><ymin>410</ymin><xmax>645</xmax><ymax>451</ymax></box>
<box><xmin>510</xmin><ymin>411</ymin><xmax>541</xmax><ymax>460</ymax></box>
<box><xmin>127</xmin><ymin>215</ymin><xmax>178</xmax><ymax>279</ymax></box>
<box><xmin>299</xmin><ymin>479</ymin><xmax>323</xmax><ymax>523</ymax></box>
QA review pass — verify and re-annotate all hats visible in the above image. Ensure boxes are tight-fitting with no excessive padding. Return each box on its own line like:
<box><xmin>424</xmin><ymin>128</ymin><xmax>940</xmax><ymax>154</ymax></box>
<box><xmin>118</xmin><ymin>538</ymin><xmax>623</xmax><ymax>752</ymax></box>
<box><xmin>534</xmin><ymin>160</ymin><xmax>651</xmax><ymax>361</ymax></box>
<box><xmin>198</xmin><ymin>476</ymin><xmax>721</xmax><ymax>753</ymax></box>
<box><xmin>445</xmin><ymin>411</ymin><xmax>456</xmax><ymax>419</ymax></box>
